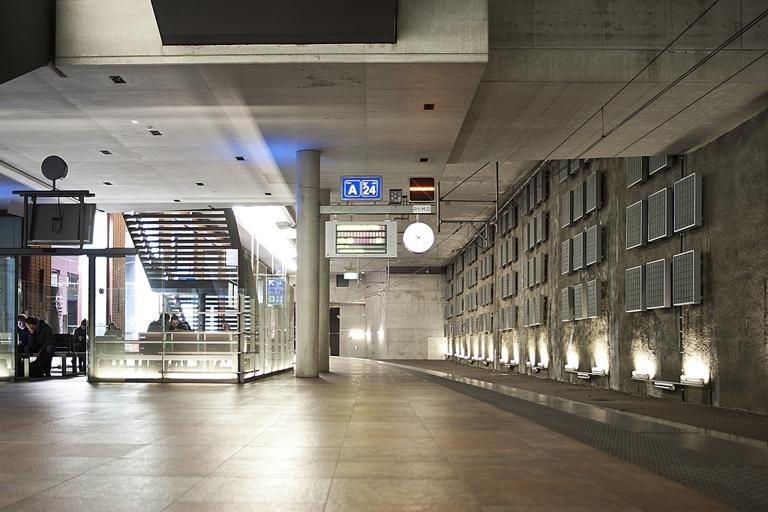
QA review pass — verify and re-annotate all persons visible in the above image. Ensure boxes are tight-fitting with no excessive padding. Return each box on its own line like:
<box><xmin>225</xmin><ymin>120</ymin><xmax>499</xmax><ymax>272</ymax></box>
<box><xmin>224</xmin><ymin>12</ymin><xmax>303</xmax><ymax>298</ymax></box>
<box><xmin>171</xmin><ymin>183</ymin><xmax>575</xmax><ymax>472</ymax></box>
<box><xmin>147</xmin><ymin>312</ymin><xmax>190</xmax><ymax>368</ymax></box>
<box><xmin>73</xmin><ymin>318</ymin><xmax>86</xmax><ymax>376</ymax></box>
<box><xmin>104</xmin><ymin>323</ymin><xmax>122</xmax><ymax>366</ymax></box>
<box><xmin>12</xmin><ymin>313</ymin><xmax>57</xmax><ymax>378</ymax></box>
<box><xmin>223</xmin><ymin>323</ymin><xmax>230</xmax><ymax>331</ymax></box>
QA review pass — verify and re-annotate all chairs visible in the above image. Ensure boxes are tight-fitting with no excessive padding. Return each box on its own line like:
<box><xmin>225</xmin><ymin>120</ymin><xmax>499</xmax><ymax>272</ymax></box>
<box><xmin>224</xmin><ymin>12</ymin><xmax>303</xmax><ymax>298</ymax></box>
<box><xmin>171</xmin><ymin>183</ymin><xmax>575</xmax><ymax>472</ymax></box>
<box><xmin>137</xmin><ymin>331</ymin><xmax>235</xmax><ymax>361</ymax></box>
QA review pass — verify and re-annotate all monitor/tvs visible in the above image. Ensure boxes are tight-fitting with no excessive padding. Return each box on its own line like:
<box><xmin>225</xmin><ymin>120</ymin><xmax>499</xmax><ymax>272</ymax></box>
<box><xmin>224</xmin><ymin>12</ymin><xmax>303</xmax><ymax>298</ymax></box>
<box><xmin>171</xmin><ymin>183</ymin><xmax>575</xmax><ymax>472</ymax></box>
<box><xmin>26</xmin><ymin>202</ymin><xmax>96</xmax><ymax>244</ymax></box>
<box><xmin>325</xmin><ymin>220</ymin><xmax>398</xmax><ymax>258</ymax></box>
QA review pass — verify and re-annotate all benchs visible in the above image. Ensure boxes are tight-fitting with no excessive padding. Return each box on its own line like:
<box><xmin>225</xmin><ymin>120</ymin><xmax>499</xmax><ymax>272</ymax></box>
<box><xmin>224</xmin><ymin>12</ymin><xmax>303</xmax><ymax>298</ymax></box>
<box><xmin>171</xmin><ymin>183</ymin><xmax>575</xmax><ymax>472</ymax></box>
<box><xmin>16</xmin><ymin>333</ymin><xmax>87</xmax><ymax>377</ymax></box>
